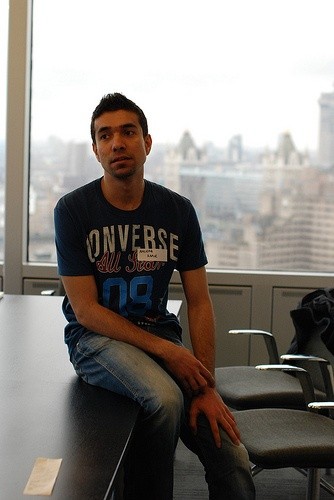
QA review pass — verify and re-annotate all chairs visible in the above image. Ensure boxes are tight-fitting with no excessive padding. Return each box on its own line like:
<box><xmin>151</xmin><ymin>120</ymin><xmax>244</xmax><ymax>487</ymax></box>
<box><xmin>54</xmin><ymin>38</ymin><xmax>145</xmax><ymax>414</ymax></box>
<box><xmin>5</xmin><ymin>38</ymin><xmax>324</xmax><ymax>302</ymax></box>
<box><xmin>230</xmin><ymin>366</ymin><xmax>334</xmax><ymax>500</ymax></box>
<box><xmin>215</xmin><ymin>315</ymin><xmax>334</xmax><ymax>416</ymax></box>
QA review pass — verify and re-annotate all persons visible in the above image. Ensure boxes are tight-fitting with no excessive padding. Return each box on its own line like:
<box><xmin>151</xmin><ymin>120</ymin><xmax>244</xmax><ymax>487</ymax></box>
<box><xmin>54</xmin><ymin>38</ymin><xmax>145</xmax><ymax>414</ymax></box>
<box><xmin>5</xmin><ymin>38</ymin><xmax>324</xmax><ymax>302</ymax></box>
<box><xmin>53</xmin><ymin>91</ymin><xmax>260</xmax><ymax>500</ymax></box>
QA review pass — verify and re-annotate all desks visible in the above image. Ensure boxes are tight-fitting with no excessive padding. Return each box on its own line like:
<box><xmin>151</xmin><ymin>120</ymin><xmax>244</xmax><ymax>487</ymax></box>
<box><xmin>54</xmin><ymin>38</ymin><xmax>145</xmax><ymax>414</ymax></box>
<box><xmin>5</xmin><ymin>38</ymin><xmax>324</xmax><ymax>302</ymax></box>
<box><xmin>0</xmin><ymin>292</ymin><xmax>183</xmax><ymax>500</ymax></box>
